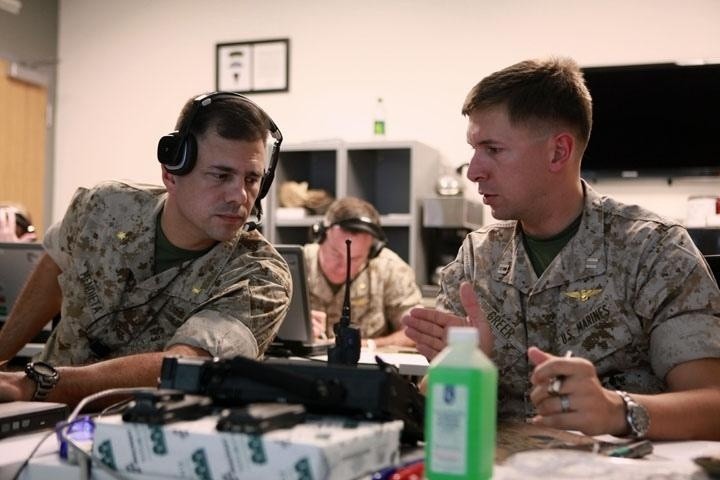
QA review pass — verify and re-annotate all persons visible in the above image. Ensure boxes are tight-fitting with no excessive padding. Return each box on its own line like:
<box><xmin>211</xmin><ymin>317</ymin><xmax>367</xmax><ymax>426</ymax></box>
<box><xmin>304</xmin><ymin>197</ymin><xmax>425</xmax><ymax>351</ymax></box>
<box><xmin>0</xmin><ymin>92</ymin><xmax>295</xmax><ymax>416</ymax></box>
<box><xmin>403</xmin><ymin>59</ymin><xmax>719</xmax><ymax>441</ymax></box>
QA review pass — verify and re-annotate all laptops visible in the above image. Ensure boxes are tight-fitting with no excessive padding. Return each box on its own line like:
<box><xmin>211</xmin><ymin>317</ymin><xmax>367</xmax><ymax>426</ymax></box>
<box><xmin>265</xmin><ymin>245</ymin><xmax>335</xmax><ymax>357</ymax></box>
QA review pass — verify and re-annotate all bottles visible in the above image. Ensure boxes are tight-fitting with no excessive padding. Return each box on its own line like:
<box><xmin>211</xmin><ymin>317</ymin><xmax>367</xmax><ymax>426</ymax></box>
<box><xmin>374</xmin><ymin>98</ymin><xmax>386</xmax><ymax>143</ymax></box>
<box><xmin>422</xmin><ymin>325</ymin><xmax>498</xmax><ymax>480</ymax></box>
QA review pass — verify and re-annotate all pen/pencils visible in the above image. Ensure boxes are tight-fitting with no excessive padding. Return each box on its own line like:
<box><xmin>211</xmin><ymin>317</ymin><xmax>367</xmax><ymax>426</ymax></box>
<box><xmin>608</xmin><ymin>439</ymin><xmax>654</xmax><ymax>458</ymax></box>
<box><xmin>547</xmin><ymin>350</ymin><xmax>575</xmax><ymax>397</ymax></box>
<box><xmin>372</xmin><ymin>458</ymin><xmax>425</xmax><ymax>480</ymax></box>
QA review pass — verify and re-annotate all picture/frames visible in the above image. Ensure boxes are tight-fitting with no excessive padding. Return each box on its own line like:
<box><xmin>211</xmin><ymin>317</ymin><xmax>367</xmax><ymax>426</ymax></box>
<box><xmin>215</xmin><ymin>37</ymin><xmax>289</xmax><ymax>96</ymax></box>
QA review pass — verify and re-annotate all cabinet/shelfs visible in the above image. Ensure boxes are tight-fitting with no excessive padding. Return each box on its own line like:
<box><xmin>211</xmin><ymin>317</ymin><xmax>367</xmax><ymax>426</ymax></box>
<box><xmin>264</xmin><ymin>145</ymin><xmax>412</xmax><ymax>294</ymax></box>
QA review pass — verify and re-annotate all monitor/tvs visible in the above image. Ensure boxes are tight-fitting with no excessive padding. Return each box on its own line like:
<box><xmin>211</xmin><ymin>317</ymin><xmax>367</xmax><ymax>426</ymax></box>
<box><xmin>578</xmin><ymin>64</ymin><xmax>720</xmax><ymax>177</ymax></box>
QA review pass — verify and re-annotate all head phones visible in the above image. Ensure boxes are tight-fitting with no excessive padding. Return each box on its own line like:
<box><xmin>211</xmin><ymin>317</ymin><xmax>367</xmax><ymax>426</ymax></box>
<box><xmin>309</xmin><ymin>215</ymin><xmax>388</xmax><ymax>260</ymax></box>
<box><xmin>157</xmin><ymin>90</ymin><xmax>283</xmax><ymax>199</ymax></box>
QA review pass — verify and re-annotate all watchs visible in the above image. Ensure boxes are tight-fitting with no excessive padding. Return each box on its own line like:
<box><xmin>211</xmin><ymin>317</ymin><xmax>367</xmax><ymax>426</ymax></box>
<box><xmin>616</xmin><ymin>390</ymin><xmax>651</xmax><ymax>442</ymax></box>
<box><xmin>25</xmin><ymin>361</ymin><xmax>60</xmax><ymax>402</ymax></box>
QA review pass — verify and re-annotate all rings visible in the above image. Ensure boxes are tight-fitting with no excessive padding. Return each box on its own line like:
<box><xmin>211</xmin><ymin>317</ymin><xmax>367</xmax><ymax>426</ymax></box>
<box><xmin>561</xmin><ymin>396</ymin><xmax>570</xmax><ymax>412</ymax></box>
<box><xmin>548</xmin><ymin>379</ymin><xmax>558</xmax><ymax>396</ymax></box>
